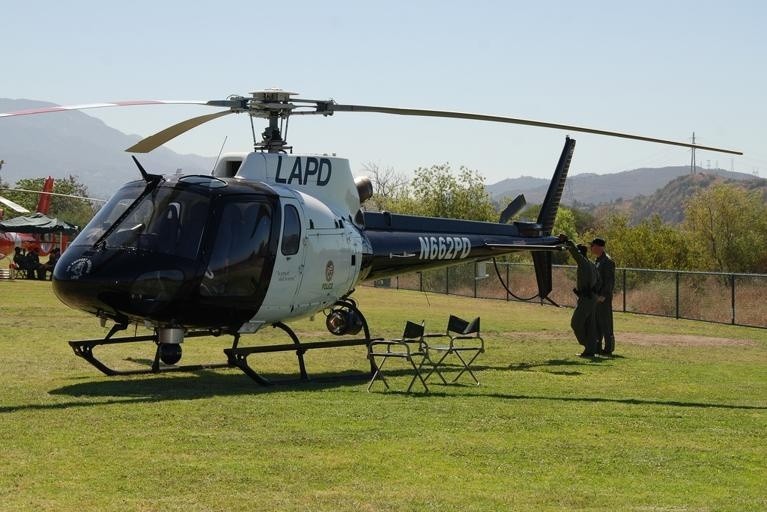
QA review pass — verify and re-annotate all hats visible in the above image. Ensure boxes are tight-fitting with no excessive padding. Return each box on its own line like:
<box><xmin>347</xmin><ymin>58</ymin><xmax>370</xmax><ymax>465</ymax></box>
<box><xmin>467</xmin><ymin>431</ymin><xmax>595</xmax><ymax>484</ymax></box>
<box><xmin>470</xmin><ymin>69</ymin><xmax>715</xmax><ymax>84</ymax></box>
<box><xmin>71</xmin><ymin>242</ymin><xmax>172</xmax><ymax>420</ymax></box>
<box><xmin>587</xmin><ymin>238</ymin><xmax>605</xmax><ymax>247</ymax></box>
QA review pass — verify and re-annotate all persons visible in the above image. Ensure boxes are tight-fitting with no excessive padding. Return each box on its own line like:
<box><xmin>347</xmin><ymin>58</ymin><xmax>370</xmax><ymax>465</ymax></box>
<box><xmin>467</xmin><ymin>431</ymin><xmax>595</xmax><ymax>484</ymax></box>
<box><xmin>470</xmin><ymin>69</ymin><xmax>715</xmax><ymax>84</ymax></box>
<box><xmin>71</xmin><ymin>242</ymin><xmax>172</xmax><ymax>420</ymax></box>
<box><xmin>35</xmin><ymin>248</ymin><xmax>60</xmax><ymax>272</ymax></box>
<box><xmin>46</xmin><ymin>248</ymin><xmax>56</xmax><ymax>265</ymax></box>
<box><xmin>558</xmin><ymin>232</ymin><xmax>603</xmax><ymax>358</ymax></box>
<box><xmin>589</xmin><ymin>238</ymin><xmax>616</xmax><ymax>356</ymax></box>
<box><xmin>26</xmin><ymin>247</ymin><xmax>46</xmax><ymax>281</ymax></box>
<box><xmin>13</xmin><ymin>246</ymin><xmax>39</xmax><ymax>280</ymax></box>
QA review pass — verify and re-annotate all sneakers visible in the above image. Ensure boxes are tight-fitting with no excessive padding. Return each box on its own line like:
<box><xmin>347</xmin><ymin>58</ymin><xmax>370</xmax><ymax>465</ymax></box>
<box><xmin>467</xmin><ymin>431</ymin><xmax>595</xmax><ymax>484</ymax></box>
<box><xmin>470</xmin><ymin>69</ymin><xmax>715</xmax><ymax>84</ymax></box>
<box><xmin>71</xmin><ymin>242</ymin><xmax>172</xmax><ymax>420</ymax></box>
<box><xmin>576</xmin><ymin>349</ymin><xmax>612</xmax><ymax>357</ymax></box>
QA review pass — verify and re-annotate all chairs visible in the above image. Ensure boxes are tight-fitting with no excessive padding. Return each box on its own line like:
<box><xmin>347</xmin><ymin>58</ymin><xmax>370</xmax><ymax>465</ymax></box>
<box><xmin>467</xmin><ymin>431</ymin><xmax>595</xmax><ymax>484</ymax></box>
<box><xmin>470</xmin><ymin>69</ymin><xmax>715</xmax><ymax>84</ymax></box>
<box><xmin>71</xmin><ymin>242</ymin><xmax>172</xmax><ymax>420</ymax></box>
<box><xmin>154</xmin><ymin>201</ymin><xmax>270</xmax><ymax>272</ymax></box>
<box><xmin>420</xmin><ymin>315</ymin><xmax>484</xmax><ymax>387</ymax></box>
<box><xmin>13</xmin><ymin>257</ymin><xmax>31</xmax><ymax>278</ymax></box>
<box><xmin>366</xmin><ymin>320</ymin><xmax>428</xmax><ymax>395</ymax></box>
